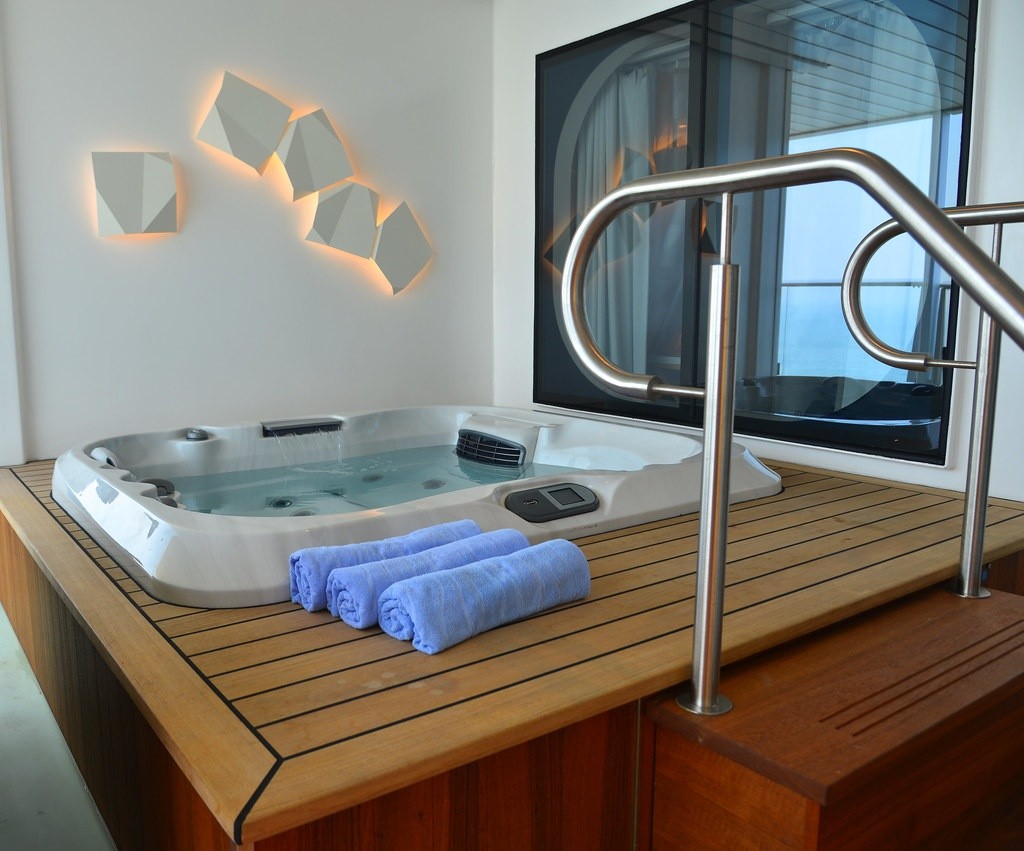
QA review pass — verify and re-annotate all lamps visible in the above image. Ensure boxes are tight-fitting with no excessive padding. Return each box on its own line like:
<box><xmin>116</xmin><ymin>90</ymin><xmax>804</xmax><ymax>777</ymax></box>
<box><xmin>91</xmin><ymin>148</ymin><xmax>179</xmax><ymax>235</ymax></box>
<box><xmin>544</xmin><ymin>130</ymin><xmax>738</xmax><ymax>282</ymax></box>
<box><xmin>195</xmin><ymin>67</ymin><xmax>434</xmax><ymax>297</ymax></box>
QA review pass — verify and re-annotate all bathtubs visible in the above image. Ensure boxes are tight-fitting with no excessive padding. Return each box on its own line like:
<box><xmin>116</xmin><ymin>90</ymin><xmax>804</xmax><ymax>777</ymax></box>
<box><xmin>49</xmin><ymin>401</ymin><xmax>787</xmax><ymax>609</ymax></box>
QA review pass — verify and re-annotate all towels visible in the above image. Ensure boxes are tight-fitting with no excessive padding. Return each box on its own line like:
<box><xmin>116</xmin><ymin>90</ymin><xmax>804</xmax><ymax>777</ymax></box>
<box><xmin>327</xmin><ymin>530</ymin><xmax>530</xmax><ymax>631</ymax></box>
<box><xmin>291</xmin><ymin>516</ymin><xmax>485</xmax><ymax>615</ymax></box>
<box><xmin>378</xmin><ymin>538</ymin><xmax>592</xmax><ymax>657</ymax></box>
